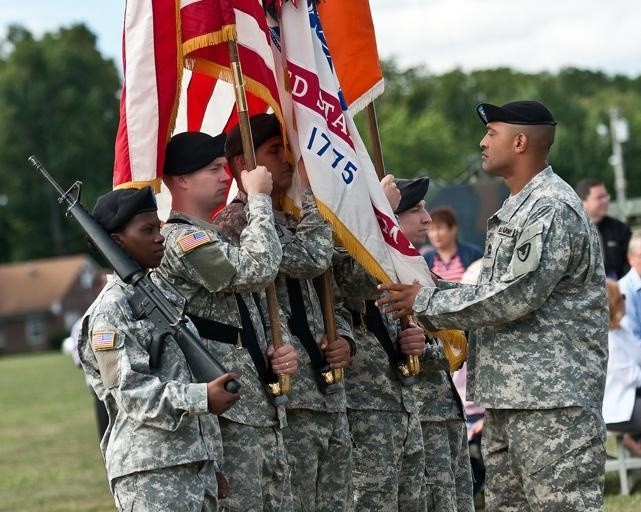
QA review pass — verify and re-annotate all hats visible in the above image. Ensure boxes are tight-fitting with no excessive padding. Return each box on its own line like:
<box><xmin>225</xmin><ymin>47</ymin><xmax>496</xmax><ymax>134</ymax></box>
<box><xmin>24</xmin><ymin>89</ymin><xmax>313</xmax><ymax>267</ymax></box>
<box><xmin>476</xmin><ymin>100</ymin><xmax>557</xmax><ymax>126</ymax></box>
<box><xmin>226</xmin><ymin>113</ymin><xmax>280</xmax><ymax>159</ymax></box>
<box><xmin>392</xmin><ymin>177</ymin><xmax>429</xmax><ymax>213</ymax></box>
<box><xmin>86</xmin><ymin>186</ymin><xmax>157</xmax><ymax>249</ymax></box>
<box><xmin>164</xmin><ymin>130</ymin><xmax>226</xmax><ymax>176</ymax></box>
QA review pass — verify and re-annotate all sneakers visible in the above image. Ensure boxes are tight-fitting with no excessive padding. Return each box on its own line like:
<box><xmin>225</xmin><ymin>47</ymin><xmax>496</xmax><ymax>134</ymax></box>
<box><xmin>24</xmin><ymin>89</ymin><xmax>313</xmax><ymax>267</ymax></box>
<box><xmin>624</xmin><ymin>433</ymin><xmax>641</xmax><ymax>455</ymax></box>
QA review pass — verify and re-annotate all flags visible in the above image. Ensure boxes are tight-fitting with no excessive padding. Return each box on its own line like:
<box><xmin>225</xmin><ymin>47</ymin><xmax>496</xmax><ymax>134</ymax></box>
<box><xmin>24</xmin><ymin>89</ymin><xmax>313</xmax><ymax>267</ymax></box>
<box><xmin>112</xmin><ymin>1</ymin><xmax>467</xmax><ymax>371</ymax></box>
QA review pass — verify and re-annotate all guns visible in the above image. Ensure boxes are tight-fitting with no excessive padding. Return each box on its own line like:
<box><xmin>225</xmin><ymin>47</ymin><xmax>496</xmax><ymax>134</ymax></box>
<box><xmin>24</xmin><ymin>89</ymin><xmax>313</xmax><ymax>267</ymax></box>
<box><xmin>28</xmin><ymin>154</ymin><xmax>243</xmax><ymax>392</ymax></box>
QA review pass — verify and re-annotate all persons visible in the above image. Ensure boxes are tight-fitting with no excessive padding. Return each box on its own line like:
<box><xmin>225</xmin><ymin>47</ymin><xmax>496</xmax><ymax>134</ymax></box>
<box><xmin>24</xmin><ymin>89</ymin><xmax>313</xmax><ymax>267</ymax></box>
<box><xmin>393</xmin><ymin>175</ymin><xmax>476</xmax><ymax>512</ymax></box>
<box><xmin>333</xmin><ymin>172</ymin><xmax>427</xmax><ymax>511</ymax></box>
<box><xmin>79</xmin><ymin>185</ymin><xmax>243</xmax><ymax>512</ymax></box>
<box><xmin>148</xmin><ymin>131</ymin><xmax>298</xmax><ymax>511</ymax></box>
<box><xmin>578</xmin><ymin>179</ymin><xmax>641</xmax><ymax>453</ymax></box>
<box><xmin>215</xmin><ymin>111</ymin><xmax>354</xmax><ymax>512</ymax></box>
<box><xmin>375</xmin><ymin>101</ymin><xmax>610</xmax><ymax>511</ymax></box>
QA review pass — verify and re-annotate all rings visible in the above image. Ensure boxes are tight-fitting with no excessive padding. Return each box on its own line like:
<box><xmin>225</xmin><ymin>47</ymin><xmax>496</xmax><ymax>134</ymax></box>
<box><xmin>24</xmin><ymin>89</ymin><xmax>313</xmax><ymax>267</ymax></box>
<box><xmin>286</xmin><ymin>362</ymin><xmax>289</xmax><ymax>367</ymax></box>
